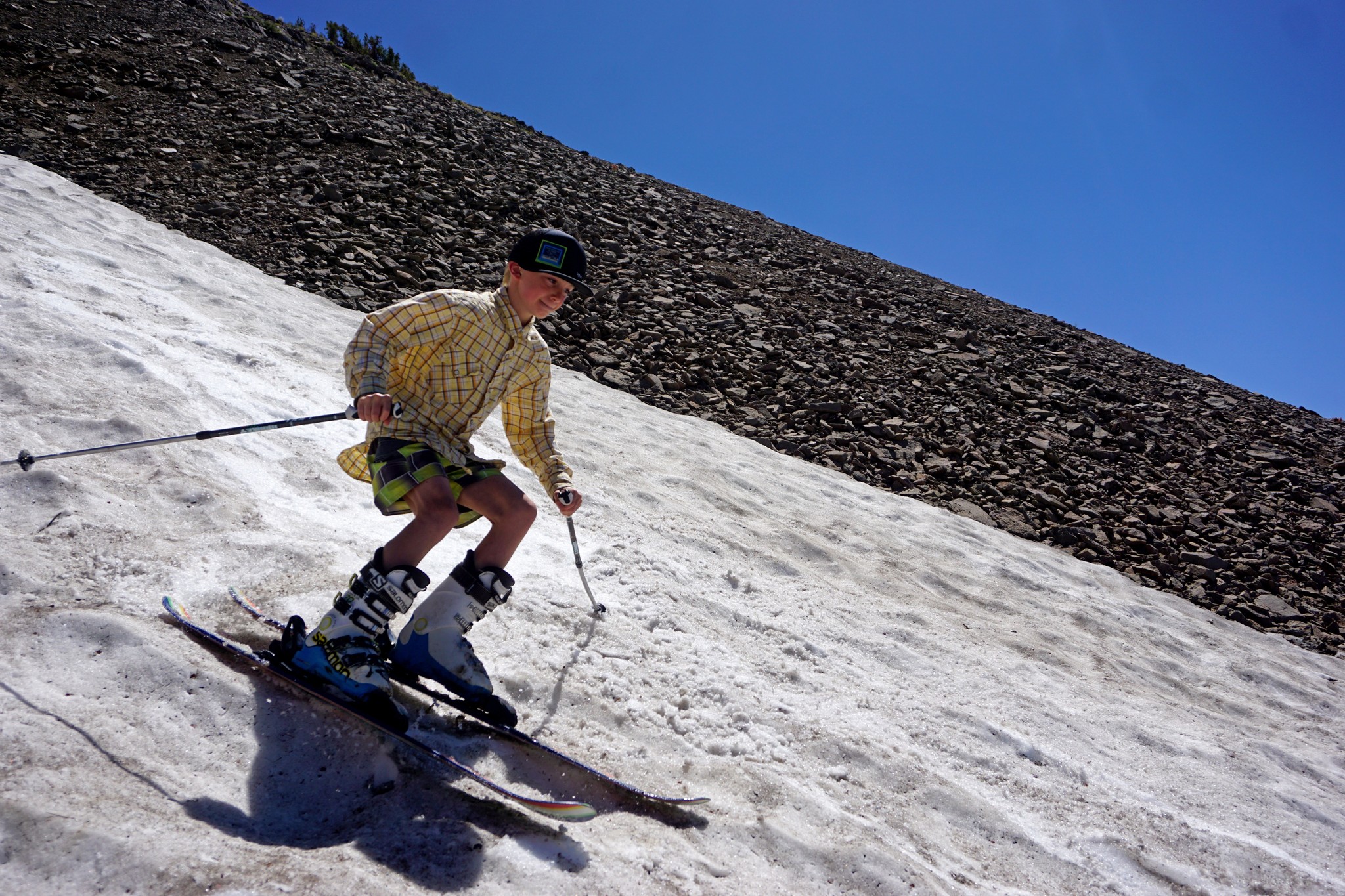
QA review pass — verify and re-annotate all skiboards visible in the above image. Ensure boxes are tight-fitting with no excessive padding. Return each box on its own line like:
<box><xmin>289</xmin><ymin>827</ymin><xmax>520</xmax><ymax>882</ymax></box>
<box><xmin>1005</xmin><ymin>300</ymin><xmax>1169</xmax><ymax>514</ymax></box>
<box><xmin>161</xmin><ymin>587</ymin><xmax>711</xmax><ymax>823</ymax></box>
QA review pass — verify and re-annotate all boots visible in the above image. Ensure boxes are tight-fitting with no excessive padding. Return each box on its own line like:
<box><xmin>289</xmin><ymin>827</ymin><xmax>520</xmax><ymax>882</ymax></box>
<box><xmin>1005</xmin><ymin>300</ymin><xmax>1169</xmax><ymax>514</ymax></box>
<box><xmin>393</xmin><ymin>549</ymin><xmax>515</xmax><ymax>698</ymax></box>
<box><xmin>290</xmin><ymin>547</ymin><xmax>430</xmax><ymax>698</ymax></box>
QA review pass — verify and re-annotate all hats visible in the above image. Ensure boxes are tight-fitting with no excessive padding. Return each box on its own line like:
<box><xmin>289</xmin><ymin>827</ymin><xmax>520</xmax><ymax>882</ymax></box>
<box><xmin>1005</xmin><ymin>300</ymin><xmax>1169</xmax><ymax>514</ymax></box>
<box><xmin>507</xmin><ymin>228</ymin><xmax>594</xmax><ymax>297</ymax></box>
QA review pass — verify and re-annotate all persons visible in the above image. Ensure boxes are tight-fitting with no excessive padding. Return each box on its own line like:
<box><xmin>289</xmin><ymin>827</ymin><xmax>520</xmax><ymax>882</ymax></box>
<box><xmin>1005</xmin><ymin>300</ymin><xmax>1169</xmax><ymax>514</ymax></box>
<box><xmin>294</xmin><ymin>229</ymin><xmax>594</xmax><ymax>706</ymax></box>
